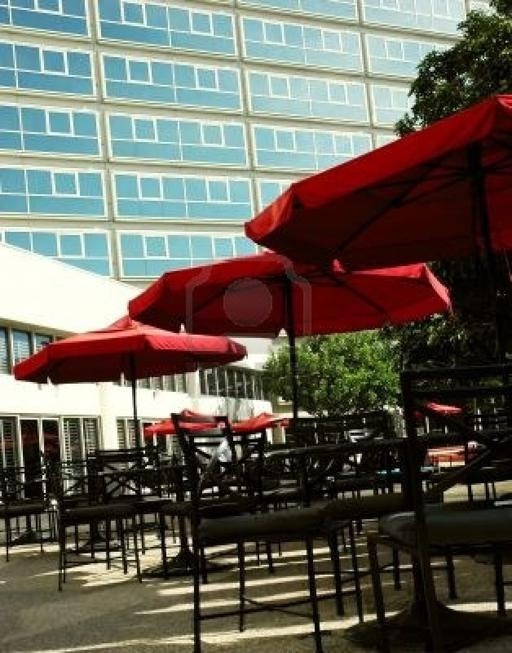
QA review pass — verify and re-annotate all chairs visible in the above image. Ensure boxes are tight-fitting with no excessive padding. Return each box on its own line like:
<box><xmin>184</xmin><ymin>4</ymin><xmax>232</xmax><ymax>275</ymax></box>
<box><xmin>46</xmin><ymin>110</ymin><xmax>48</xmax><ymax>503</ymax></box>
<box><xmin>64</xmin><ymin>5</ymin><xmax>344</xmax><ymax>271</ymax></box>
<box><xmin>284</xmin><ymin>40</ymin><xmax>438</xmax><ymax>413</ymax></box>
<box><xmin>0</xmin><ymin>348</ymin><xmax>512</xmax><ymax>653</ymax></box>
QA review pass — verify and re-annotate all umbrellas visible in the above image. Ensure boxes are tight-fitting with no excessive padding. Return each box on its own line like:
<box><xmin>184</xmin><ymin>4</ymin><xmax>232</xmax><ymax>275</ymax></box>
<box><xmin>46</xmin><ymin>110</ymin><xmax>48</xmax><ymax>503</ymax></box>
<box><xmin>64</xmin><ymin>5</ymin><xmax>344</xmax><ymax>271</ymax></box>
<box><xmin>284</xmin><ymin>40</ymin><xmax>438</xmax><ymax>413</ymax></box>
<box><xmin>144</xmin><ymin>408</ymin><xmax>230</xmax><ymax>437</ymax></box>
<box><xmin>13</xmin><ymin>315</ymin><xmax>248</xmax><ymax>500</ymax></box>
<box><xmin>231</xmin><ymin>412</ymin><xmax>291</xmax><ymax>434</ymax></box>
<box><xmin>415</xmin><ymin>401</ymin><xmax>462</xmax><ymax>417</ymax></box>
<box><xmin>245</xmin><ymin>90</ymin><xmax>512</xmax><ymax>520</ymax></box>
<box><xmin>128</xmin><ymin>247</ymin><xmax>452</xmax><ymax>508</ymax></box>
